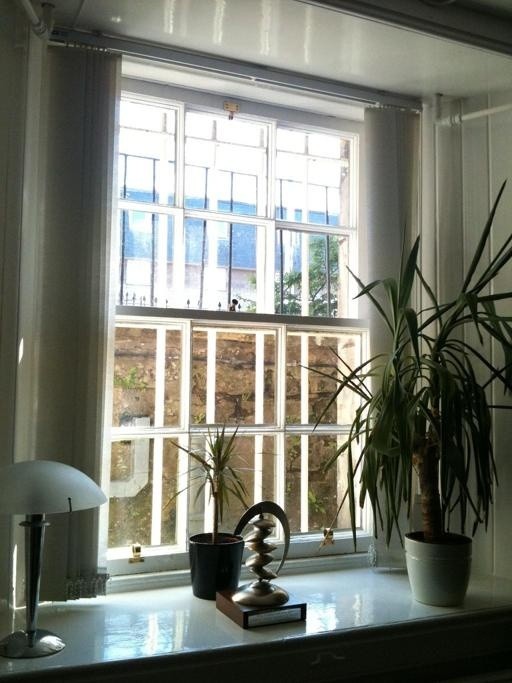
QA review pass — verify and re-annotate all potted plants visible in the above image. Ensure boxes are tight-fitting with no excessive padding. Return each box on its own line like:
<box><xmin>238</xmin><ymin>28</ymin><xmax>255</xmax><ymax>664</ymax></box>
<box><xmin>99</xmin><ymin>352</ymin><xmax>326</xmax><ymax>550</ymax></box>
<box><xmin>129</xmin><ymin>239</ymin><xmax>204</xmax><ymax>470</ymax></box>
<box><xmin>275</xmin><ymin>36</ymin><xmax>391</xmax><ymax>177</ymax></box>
<box><xmin>161</xmin><ymin>419</ymin><xmax>250</xmax><ymax>602</ymax></box>
<box><xmin>314</xmin><ymin>175</ymin><xmax>511</xmax><ymax>609</ymax></box>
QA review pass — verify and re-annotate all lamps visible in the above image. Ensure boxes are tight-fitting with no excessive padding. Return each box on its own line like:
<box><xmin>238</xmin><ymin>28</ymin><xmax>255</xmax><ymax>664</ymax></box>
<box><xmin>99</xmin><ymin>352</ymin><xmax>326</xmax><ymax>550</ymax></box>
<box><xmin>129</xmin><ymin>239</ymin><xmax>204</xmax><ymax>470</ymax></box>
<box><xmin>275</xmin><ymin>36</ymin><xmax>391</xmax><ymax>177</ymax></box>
<box><xmin>0</xmin><ymin>458</ymin><xmax>108</xmax><ymax>660</ymax></box>
